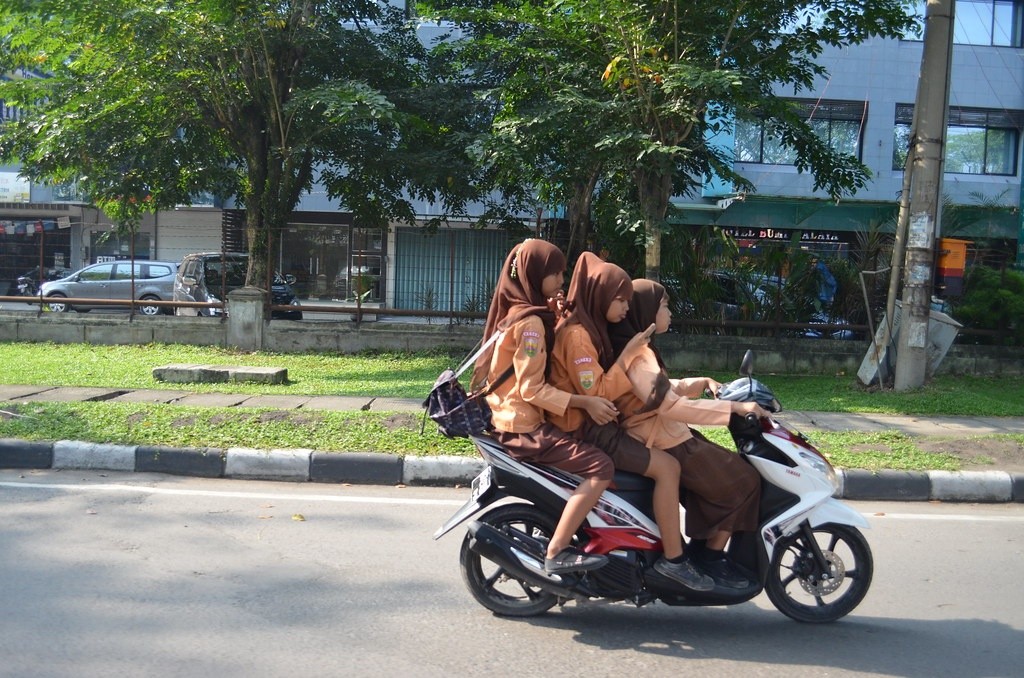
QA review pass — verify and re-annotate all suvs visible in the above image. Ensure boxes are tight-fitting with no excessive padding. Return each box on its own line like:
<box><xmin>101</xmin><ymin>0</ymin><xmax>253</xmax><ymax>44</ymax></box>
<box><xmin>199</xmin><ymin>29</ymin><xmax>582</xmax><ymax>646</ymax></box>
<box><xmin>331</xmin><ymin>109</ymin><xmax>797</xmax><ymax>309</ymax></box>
<box><xmin>172</xmin><ymin>248</ymin><xmax>303</xmax><ymax>322</ymax></box>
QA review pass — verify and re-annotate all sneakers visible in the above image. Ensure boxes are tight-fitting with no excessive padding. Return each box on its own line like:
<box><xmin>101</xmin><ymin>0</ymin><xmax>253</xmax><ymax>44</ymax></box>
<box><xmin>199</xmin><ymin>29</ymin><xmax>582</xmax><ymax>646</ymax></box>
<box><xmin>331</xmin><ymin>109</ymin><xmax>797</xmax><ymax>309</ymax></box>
<box><xmin>653</xmin><ymin>553</ymin><xmax>716</xmax><ymax>592</ymax></box>
<box><xmin>544</xmin><ymin>546</ymin><xmax>609</xmax><ymax>573</ymax></box>
<box><xmin>693</xmin><ymin>552</ymin><xmax>749</xmax><ymax>589</ymax></box>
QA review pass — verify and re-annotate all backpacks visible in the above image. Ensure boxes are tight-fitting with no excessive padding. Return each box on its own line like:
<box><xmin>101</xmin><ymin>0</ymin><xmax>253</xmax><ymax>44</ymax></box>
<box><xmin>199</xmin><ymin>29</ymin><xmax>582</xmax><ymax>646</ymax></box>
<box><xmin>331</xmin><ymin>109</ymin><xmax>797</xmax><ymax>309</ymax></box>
<box><xmin>419</xmin><ymin>370</ymin><xmax>497</xmax><ymax>441</ymax></box>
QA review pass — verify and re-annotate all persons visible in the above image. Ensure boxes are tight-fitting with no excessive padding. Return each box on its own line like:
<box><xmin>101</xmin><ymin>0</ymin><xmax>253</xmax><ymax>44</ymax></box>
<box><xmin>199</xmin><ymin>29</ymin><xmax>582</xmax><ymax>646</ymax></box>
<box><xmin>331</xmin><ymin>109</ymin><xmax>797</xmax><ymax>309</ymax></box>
<box><xmin>470</xmin><ymin>238</ymin><xmax>620</xmax><ymax>575</ymax></box>
<box><xmin>546</xmin><ymin>251</ymin><xmax>716</xmax><ymax>591</ymax></box>
<box><xmin>608</xmin><ymin>279</ymin><xmax>773</xmax><ymax>589</ymax></box>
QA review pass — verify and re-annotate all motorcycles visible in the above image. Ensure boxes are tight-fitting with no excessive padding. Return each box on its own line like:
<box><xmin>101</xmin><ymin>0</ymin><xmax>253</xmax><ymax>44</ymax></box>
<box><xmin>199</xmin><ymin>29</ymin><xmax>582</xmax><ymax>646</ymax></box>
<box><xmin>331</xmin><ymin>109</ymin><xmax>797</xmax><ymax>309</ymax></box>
<box><xmin>427</xmin><ymin>352</ymin><xmax>875</xmax><ymax>627</ymax></box>
<box><xmin>17</xmin><ymin>268</ymin><xmax>65</xmax><ymax>305</ymax></box>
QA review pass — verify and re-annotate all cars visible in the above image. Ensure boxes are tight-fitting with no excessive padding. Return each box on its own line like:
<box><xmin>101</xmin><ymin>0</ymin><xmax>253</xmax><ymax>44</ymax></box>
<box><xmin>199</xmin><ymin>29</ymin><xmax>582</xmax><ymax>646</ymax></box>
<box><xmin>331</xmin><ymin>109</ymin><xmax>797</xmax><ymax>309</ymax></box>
<box><xmin>663</xmin><ymin>267</ymin><xmax>861</xmax><ymax>339</ymax></box>
<box><xmin>36</xmin><ymin>257</ymin><xmax>184</xmax><ymax>316</ymax></box>
